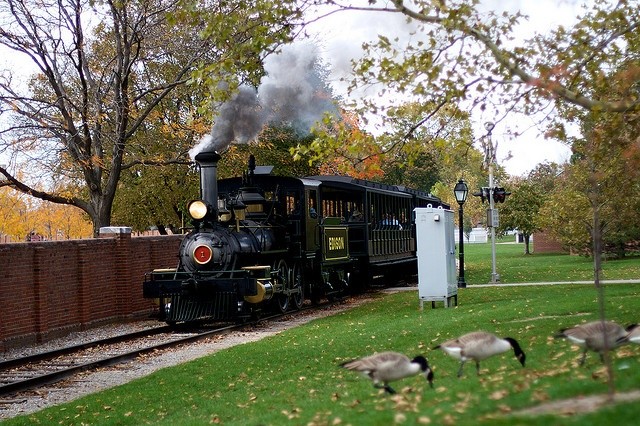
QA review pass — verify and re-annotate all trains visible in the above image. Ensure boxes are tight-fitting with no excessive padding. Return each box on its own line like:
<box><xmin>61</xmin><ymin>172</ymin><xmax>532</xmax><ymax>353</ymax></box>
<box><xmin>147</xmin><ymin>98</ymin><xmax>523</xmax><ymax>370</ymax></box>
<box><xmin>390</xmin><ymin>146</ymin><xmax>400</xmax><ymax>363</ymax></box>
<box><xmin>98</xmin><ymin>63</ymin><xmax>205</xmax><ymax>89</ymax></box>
<box><xmin>143</xmin><ymin>151</ymin><xmax>450</xmax><ymax>323</ymax></box>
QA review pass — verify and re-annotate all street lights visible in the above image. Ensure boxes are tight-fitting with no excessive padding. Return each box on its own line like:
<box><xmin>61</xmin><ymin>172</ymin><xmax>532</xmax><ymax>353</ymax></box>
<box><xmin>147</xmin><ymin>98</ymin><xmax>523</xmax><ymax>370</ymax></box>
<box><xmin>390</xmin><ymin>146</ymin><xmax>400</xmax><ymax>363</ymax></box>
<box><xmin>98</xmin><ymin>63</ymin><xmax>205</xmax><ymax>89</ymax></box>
<box><xmin>454</xmin><ymin>179</ymin><xmax>468</xmax><ymax>288</ymax></box>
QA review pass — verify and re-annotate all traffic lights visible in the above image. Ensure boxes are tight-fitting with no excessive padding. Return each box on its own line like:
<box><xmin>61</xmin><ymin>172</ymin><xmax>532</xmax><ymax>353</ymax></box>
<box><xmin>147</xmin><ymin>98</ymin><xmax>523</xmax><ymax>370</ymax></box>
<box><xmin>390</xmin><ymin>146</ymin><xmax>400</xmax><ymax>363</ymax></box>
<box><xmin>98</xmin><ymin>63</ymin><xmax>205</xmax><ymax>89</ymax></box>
<box><xmin>475</xmin><ymin>186</ymin><xmax>489</xmax><ymax>202</ymax></box>
<box><xmin>493</xmin><ymin>187</ymin><xmax>512</xmax><ymax>203</ymax></box>
